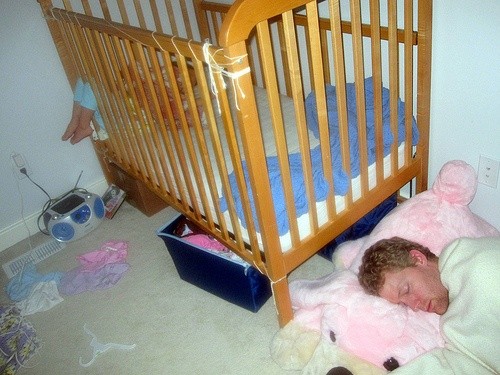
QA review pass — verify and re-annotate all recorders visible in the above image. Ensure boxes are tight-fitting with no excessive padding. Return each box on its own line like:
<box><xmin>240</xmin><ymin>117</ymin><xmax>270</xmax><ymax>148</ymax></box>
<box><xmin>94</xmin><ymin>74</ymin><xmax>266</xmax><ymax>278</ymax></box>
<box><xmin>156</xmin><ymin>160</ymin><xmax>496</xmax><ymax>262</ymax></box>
<box><xmin>43</xmin><ymin>170</ymin><xmax>106</xmax><ymax>243</ymax></box>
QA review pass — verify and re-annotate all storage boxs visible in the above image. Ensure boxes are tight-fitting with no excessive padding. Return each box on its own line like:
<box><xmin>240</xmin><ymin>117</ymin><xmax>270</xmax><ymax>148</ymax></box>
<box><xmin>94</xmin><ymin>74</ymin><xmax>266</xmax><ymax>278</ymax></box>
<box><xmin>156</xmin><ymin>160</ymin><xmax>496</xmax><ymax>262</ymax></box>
<box><xmin>156</xmin><ymin>213</ymin><xmax>271</xmax><ymax>316</ymax></box>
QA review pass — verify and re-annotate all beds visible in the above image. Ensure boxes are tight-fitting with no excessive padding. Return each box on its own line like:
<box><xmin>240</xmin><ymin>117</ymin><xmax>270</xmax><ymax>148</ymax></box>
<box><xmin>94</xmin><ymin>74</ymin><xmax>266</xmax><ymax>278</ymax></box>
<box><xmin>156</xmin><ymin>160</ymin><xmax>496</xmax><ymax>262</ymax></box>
<box><xmin>37</xmin><ymin>1</ymin><xmax>431</xmax><ymax>329</ymax></box>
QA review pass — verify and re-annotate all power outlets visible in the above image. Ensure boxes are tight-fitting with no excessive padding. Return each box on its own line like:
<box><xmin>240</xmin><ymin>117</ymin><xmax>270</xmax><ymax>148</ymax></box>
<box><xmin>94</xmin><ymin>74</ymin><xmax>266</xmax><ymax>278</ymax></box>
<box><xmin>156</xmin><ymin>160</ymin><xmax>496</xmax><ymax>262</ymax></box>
<box><xmin>477</xmin><ymin>153</ymin><xmax>500</xmax><ymax>189</ymax></box>
<box><xmin>10</xmin><ymin>151</ymin><xmax>34</xmax><ymax>180</ymax></box>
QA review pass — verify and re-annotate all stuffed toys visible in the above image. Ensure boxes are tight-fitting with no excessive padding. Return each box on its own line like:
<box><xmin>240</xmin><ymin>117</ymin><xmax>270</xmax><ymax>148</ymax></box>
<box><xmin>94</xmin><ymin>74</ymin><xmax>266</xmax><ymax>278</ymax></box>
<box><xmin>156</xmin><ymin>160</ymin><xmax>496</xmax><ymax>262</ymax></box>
<box><xmin>269</xmin><ymin>159</ymin><xmax>499</xmax><ymax>373</ymax></box>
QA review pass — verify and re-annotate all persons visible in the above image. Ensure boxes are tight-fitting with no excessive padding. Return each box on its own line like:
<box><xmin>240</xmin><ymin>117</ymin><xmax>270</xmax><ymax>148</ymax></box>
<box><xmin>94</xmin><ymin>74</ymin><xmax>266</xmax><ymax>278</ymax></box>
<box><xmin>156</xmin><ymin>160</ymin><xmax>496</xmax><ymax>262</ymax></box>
<box><xmin>61</xmin><ymin>59</ymin><xmax>199</xmax><ymax>145</ymax></box>
<box><xmin>357</xmin><ymin>235</ymin><xmax>499</xmax><ymax>374</ymax></box>
